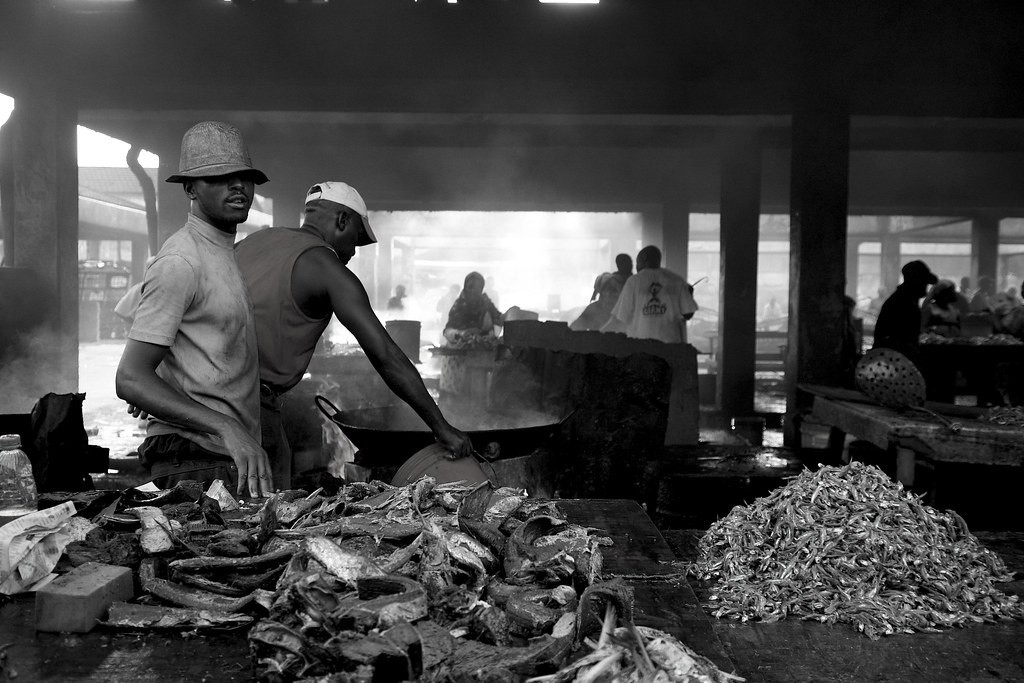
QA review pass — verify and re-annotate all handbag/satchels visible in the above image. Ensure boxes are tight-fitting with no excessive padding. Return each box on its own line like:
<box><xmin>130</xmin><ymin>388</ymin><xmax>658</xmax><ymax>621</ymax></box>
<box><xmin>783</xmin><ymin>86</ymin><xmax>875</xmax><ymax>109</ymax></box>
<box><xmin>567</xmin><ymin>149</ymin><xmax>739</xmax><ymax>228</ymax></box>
<box><xmin>25</xmin><ymin>391</ymin><xmax>95</xmax><ymax>491</ymax></box>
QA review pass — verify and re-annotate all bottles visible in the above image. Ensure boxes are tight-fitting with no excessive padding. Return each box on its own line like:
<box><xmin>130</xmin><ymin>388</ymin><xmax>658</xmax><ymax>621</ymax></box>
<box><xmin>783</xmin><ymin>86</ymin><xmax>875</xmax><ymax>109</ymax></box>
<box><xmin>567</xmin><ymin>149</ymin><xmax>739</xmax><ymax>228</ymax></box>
<box><xmin>0</xmin><ymin>433</ymin><xmax>39</xmax><ymax>527</ymax></box>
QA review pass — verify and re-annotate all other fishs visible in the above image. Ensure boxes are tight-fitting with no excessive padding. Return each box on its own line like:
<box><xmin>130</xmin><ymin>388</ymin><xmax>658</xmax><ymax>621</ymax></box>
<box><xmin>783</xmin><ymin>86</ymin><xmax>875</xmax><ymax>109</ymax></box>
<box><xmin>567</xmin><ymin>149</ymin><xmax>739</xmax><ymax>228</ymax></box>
<box><xmin>102</xmin><ymin>472</ymin><xmax>747</xmax><ymax>683</ymax></box>
<box><xmin>687</xmin><ymin>457</ymin><xmax>1023</xmax><ymax>639</ymax></box>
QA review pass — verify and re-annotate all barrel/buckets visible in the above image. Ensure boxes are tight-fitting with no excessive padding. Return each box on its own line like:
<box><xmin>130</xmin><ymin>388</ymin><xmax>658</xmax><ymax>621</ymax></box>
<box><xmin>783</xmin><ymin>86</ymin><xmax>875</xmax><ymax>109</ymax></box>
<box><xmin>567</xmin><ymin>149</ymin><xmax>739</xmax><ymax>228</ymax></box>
<box><xmin>385</xmin><ymin>319</ymin><xmax>422</xmax><ymax>365</ymax></box>
<box><xmin>390</xmin><ymin>440</ymin><xmax>501</xmax><ymax>504</ymax></box>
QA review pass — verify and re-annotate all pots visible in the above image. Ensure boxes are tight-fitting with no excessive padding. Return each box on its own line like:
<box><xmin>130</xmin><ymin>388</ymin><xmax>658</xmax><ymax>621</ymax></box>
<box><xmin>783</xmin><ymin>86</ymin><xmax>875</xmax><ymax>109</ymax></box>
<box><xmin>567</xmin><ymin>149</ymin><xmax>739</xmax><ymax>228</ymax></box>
<box><xmin>313</xmin><ymin>395</ymin><xmax>582</xmax><ymax>464</ymax></box>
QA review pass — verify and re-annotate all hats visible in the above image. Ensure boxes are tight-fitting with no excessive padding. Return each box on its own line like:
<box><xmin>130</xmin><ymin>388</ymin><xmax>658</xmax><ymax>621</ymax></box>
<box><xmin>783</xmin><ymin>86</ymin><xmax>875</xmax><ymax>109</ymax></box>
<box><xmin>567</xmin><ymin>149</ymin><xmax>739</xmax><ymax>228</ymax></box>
<box><xmin>305</xmin><ymin>180</ymin><xmax>376</xmax><ymax>247</ymax></box>
<box><xmin>901</xmin><ymin>260</ymin><xmax>939</xmax><ymax>286</ymax></box>
<box><xmin>165</xmin><ymin>120</ymin><xmax>269</xmax><ymax>187</ymax></box>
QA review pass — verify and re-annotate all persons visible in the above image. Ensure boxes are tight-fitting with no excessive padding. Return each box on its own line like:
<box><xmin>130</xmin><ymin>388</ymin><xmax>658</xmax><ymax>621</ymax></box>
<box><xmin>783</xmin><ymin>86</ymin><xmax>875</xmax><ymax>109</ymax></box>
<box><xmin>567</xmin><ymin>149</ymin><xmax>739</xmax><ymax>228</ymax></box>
<box><xmin>873</xmin><ymin>258</ymin><xmax>938</xmax><ymax>359</ymax></box>
<box><xmin>436</xmin><ymin>283</ymin><xmax>460</xmax><ymax>310</ymax></box>
<box><xmin>388</xmin><ymin>284</ymin><xmax>407</xmax><ymax>309</ymax></box>
<box><xmin>570</xmin><ymin>254</ymin><xmax>635</xmax><ymax>334</ymax></box>
<box><xmin>436</xmin><ymin>271</ymin><xmax>502</xmax><ymax>402</ymax></box>
<box><xmin>136</xmin><ymin>182</ymin><xmax>474</xmax><ymax>495</ymax></box>
<box><xmin>600</xmin><ymin>244</ymin><xmax>698</xmax><ymax>344</ymax></box>
<box><xmin>115</xmin><ymin>121</ymin><xmax>276</xmax><ymax>498</ymax></box>
<box><xmin>922</xmin><ymin>277</ymin><xmax>1024</xmax><ymax>326</ymax></box>
